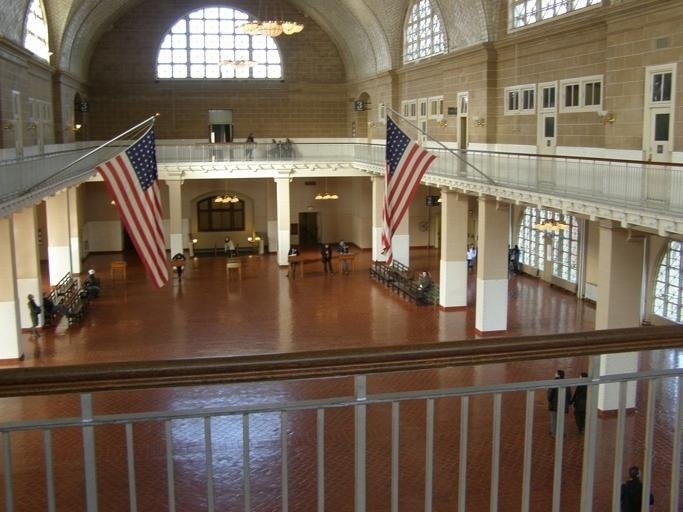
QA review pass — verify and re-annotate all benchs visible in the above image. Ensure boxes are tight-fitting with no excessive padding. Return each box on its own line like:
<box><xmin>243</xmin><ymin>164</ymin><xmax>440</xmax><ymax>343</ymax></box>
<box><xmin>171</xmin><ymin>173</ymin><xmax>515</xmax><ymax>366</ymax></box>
<box><xmin>369</xmin><ymin>259</ymin><xmax>439</xmax><ymax>306</ymax></box>
<box><xmin>46</xmin><ymin>272</ymin><xmax>88</xmax><ymax>328</ymax></box>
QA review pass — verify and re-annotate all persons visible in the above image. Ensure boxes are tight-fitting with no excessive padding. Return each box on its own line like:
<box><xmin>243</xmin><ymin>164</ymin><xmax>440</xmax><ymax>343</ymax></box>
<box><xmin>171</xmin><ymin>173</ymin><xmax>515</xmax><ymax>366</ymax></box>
<box><xmin>415</xmin><ymin>270</ymin><xmax>431</xmax><ymax>296</ymax></box>
<box><xmin>319</xmin><ymin>242</ymin><xmax>333</xmax><ymax>274</ymax></box>
<box><xmin>544</xmin><ymin>369</ymin><xmax>572</xmax><ymax>438</ymax></box>
<box><xmin>284</xmin><ymin>245</ymin><xmax>300</xmax><ymax>277</ymax></box>
<box><xmin>507</xmin><ymin>244</ymin><xmax>520</xmax><ymax>274</ymax></box>
<box><xmin>618</xmin><ymin>463</ymin><xmax>654</xmax><ymax>512</ymax></box>
<box><xmin>242</xmin><ymin>132</ymin><xmax>257</xmax><ymax>159</ymax></box>
<box><xmin>25</xmin><ymin>293</ymin><xmax>41</xmax><ymax>339</ymax></box>
<box><xmin>268</xmin><ymin>137</ymin><xmax>292</xmax><ymax>161</ymax></box>
<box><xmin>465</xmin><ymin>243</ymin><xmax>476</xmax><ymax>275</ymax></box>
<box><xmin>223</xmin><ymin>236</ymin><xmax>235</xmax><ymax>258</ymax></box>
<box><xmin>570</xmin><ymin>371</ymin><xmax>587</xmax><ymax>434</ymax></box>
<box><xmin>335</xmin><ymin>240</ymin><xmax>349</xmax><ymax>275</ymax></box>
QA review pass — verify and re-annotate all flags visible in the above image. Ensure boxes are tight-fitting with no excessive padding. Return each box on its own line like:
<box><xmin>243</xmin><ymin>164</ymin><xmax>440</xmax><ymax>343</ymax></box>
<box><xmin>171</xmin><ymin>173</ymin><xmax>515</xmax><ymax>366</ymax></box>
<box><xmin>380</xmin><ymin>114</ymin><xmax>438</xmax><ymax>267</ymax></box>
<box><xmin>92</xmin><ymin>123</ymin><xmax>170</xmax><ymax>290</ymax></box>
